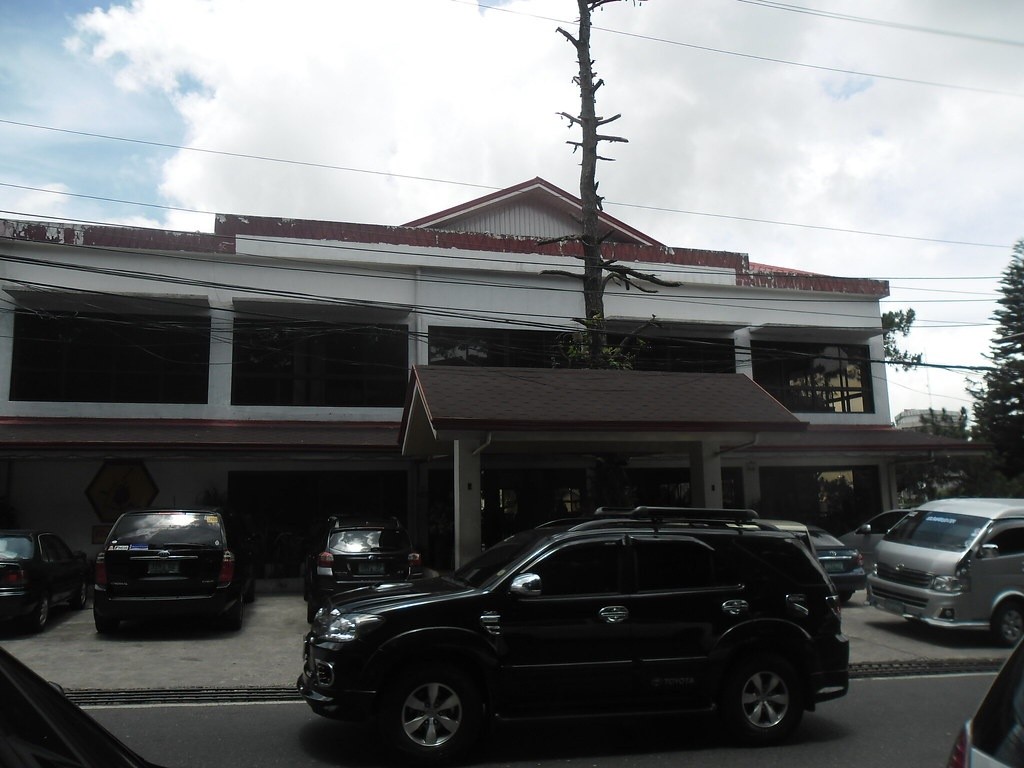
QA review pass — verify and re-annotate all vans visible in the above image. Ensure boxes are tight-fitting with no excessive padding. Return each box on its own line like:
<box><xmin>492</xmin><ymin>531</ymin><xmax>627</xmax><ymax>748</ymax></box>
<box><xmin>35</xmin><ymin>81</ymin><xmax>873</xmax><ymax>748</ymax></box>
<box><xmin>864</xmin><ymin>496</ymin><xmax>1024</xmax><ymax>648</ymax></box>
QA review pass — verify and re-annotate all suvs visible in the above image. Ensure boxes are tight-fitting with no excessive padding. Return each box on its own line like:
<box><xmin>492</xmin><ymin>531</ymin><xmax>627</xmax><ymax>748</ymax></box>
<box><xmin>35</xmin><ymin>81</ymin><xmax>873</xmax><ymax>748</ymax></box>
<box><xmin>93</xmin><ymin>508</ymin><xmax>258</xmax><ymax>634</ymax></box>
<box><xmin>295</xmin><ymin>504</ymin><xmax>851</xmax><ymax>767</ymax></box>
<box><xmin>300</xmin><ymin>514</ymin><xmax>426</xmax><ymax>625</ymax></box>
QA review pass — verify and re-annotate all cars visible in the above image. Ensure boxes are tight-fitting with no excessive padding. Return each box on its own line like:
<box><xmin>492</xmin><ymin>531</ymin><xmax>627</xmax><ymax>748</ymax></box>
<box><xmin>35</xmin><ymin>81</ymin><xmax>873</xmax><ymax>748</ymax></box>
<box><xmin>836</xmin><ymin>509</ymin><xmax>916</xmax><ymax>578</ymax></box>
<box><xmin>0</xmin><ymin>528</ymin><xmax>89</xmax><ymax>634</ymax></box>
<box><xmin>729</xmin><ymin>519</ymin><xmax>818</xmax><ymax>561</ymax></box>
<box><xmin>804</xmin><ymin>523</ymin><xmax>868</xmax><ymax>608</ymax></box>
